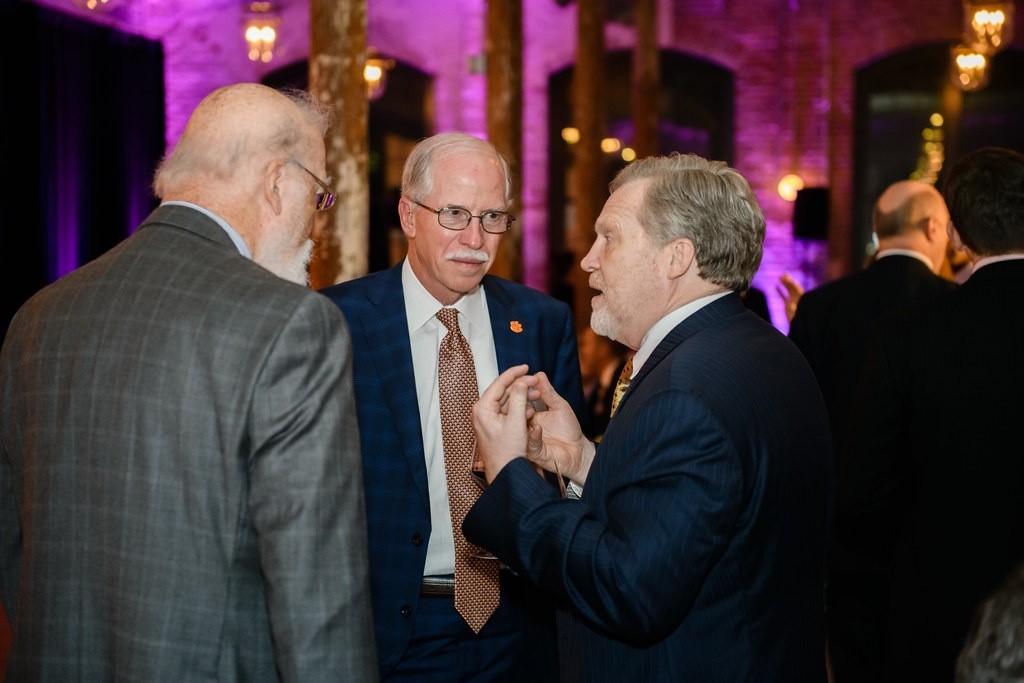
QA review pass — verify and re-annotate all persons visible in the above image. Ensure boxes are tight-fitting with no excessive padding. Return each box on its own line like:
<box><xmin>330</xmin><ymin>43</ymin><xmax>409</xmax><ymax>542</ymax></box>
<box><xmin>917</xmin><ymin>135</ymin><xmax>1024</xmax><ymax>683</ymax></box>
<box><xmin>780</xmin><ymin>178</ymin><xmax>964</xmax><ymax>683</ymax></box>
<box><xmin>1</xmin><ymin>86</ymin><xmax>380</xmax><ymax>682</ymax></box>
<box><xmin>327</xmin><ymin>130</ymin><xmax>609</xmax><ymax>682</ymax></box>
<box><xmin>463</xmin><ymin>144</ymin><xmax>840</xmax><ymax>681</ymax></box>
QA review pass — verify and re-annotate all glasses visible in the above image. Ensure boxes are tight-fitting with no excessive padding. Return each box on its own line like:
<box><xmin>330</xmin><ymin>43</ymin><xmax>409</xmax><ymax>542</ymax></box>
<box><xmin>282</xmin><ymin>158</ymin><xmax>339</xmax><ymax>212</ymax></box>
<box><xmin>404</xmin><ymin>195</ymin><xmax>517</xmax><ymax>235</ymax></box>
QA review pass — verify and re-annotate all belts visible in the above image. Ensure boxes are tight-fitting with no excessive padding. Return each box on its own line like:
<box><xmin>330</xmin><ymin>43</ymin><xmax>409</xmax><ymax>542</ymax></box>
<box><xmin>421</xmin><ymin>573</ymin><xmax>457</xmax><ymax>598</ymax></box>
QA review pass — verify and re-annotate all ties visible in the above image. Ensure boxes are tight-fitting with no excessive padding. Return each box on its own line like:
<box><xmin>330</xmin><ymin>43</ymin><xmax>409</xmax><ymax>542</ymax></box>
<box><xmin>436</xmin><ymin>308</ymin><xmax>501</xmax><ymax>635</ymax></box>
<box><xmin>610</xmin><ymin>357</ymin><xmax>634</xmax><ymax>418</ymax></box>
